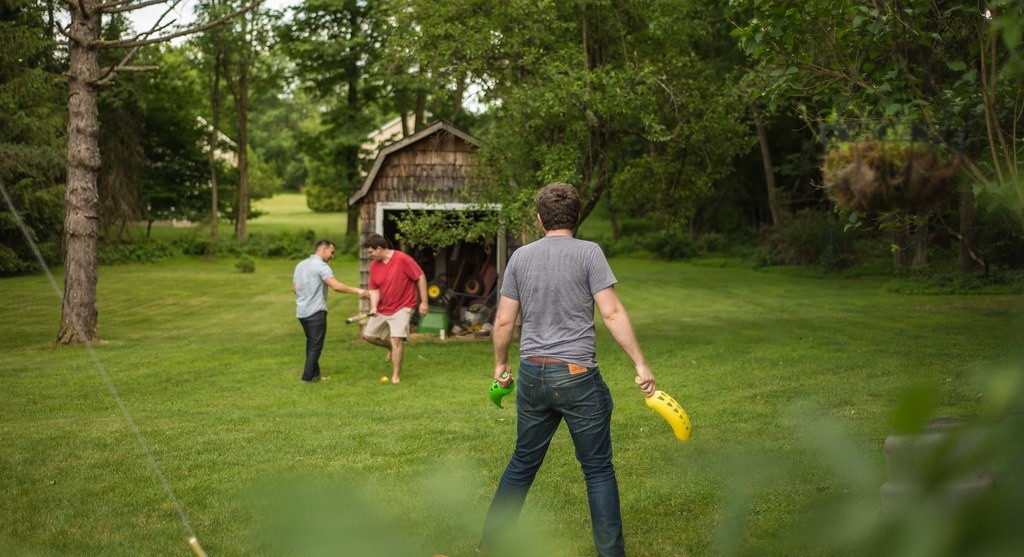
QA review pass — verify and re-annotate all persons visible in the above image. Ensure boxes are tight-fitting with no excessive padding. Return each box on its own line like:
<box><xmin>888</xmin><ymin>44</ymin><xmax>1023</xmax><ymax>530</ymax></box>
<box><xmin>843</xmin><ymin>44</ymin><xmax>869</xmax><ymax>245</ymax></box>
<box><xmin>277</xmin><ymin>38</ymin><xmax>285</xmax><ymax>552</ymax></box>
<box><xmin>474</xmin><ymin>182</ymin><xmax>656</xmax><ymax>557</ymax></box>
<box><xmin>362</xmin><ymin>233</ymin><xmax>428</xmax><ymax>385</ymax></box>
<box><xmin>293</xmin><ymin>239</ymin><xmax>370</xmax><ymax>383</ymax></box>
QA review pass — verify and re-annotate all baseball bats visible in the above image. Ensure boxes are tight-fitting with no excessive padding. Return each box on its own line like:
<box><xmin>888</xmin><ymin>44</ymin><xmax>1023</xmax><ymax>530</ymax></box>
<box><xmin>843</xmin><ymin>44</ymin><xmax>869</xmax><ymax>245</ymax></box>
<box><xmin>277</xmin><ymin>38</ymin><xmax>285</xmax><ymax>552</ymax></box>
<box><xmin>345</xmin><ymin>313</ymin><xmax>374</xmax><ymax>325</ymax></box>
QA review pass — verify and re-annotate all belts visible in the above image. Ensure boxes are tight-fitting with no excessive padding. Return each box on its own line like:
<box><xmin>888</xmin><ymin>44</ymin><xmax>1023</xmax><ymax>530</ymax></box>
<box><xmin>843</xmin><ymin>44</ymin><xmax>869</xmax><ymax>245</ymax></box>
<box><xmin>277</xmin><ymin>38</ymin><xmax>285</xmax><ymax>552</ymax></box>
<box><xmin>526</xmin><ymin>356</ymin><xmax>564</xmax><ymax>364</ymax></box>
